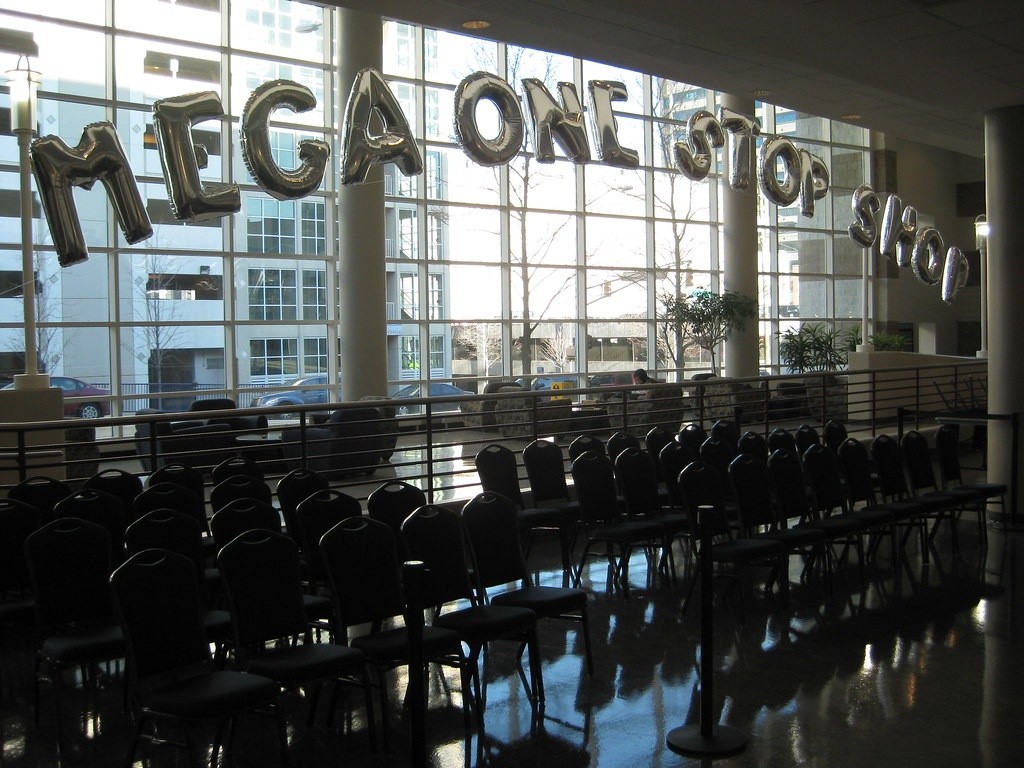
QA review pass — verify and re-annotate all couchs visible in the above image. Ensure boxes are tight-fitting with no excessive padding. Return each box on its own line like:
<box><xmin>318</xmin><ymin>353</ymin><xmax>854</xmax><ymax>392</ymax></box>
<box><xmin>459</xmin><ymin>368</ymin><xmax>850</xmax><ymax>439</ymax></box>
<box><xmin>132</xmin><ymin>395</ymin><xmax>401</xmax><ymax>479</ymax></box>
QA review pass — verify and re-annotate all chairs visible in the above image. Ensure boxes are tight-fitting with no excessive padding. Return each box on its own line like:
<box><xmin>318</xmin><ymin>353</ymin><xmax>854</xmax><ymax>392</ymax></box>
<box><xmin>0</xmin><ymin>418</ymin><xmax>1009</xmax><ymax>767</ymax></box>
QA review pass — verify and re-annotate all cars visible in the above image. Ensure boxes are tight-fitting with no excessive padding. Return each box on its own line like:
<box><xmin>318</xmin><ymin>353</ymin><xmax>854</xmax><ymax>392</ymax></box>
<box><xmin>391</xmin><ymin>383</ymin><xmax>476</xmax><ymax>413</ymax></box>
<box><xmin>2</xmin><ymin>376</ymin><xmax>112</xmax><ymax>421</ymax></box>
<box><xmin>515</xmin><ymin>377</ymin><xmax>579</xmax><ymax>403</ymax></box>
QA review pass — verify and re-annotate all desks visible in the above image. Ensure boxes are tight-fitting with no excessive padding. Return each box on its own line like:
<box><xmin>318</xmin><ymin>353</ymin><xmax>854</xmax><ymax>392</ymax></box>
<box><xmin>234</xmin><ymin>430</ymin><xmax>290</xmax><ymax>475</ymax></box>
<box><xmin>571</xmin><ymin>402</ymin><xmax>610</xmax><ymax>436</ymax></box>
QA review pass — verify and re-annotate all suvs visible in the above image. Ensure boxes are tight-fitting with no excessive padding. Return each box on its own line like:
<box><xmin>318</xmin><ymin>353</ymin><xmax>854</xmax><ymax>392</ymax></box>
<box><xmin>589</xmin><ymin>372</ymin><xmax>638</xmax><ymax>395</ymax></box>
<box><xmin>250</xmin><ymin>374</ymin><xmax>341</xmax><ymax>419</ymax></box>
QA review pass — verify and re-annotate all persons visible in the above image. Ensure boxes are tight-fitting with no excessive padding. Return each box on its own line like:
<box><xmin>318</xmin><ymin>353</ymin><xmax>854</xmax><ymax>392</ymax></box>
<box><xmin>633</xmin><ymin>369</ymin><xmax>666</xmax><ymax>385</ymax></box>
